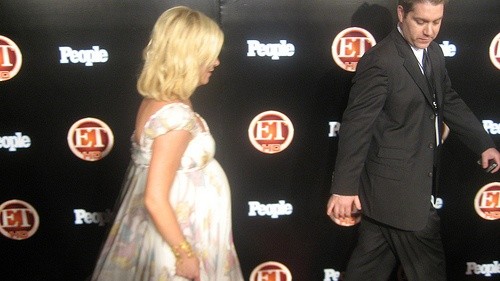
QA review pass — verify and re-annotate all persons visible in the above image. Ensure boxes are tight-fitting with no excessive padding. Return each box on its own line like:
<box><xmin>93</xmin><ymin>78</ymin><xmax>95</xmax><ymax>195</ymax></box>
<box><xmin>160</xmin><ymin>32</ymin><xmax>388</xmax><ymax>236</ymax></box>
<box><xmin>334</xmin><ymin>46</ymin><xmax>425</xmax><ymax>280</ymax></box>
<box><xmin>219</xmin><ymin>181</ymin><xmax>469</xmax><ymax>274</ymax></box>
<box><xmin>90</xmin><ymin>5</ymin><xmax>245</xmax><ymax>281</ymax></box>
<box><xmin>326</xmin><ymin>0</ymin><xmax>500</xmax><ymax>281</ymax></box>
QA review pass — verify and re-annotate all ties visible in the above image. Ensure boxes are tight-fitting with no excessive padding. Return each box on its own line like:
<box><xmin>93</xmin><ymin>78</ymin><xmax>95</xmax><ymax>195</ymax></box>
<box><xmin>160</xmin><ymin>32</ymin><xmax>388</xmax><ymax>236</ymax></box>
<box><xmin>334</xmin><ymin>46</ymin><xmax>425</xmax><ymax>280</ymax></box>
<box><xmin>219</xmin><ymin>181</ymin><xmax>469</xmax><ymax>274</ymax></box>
<box><xmin>421</xmin><ymin>48</ymin><xmax>435</xmax><ymax>95</ymax></box>
